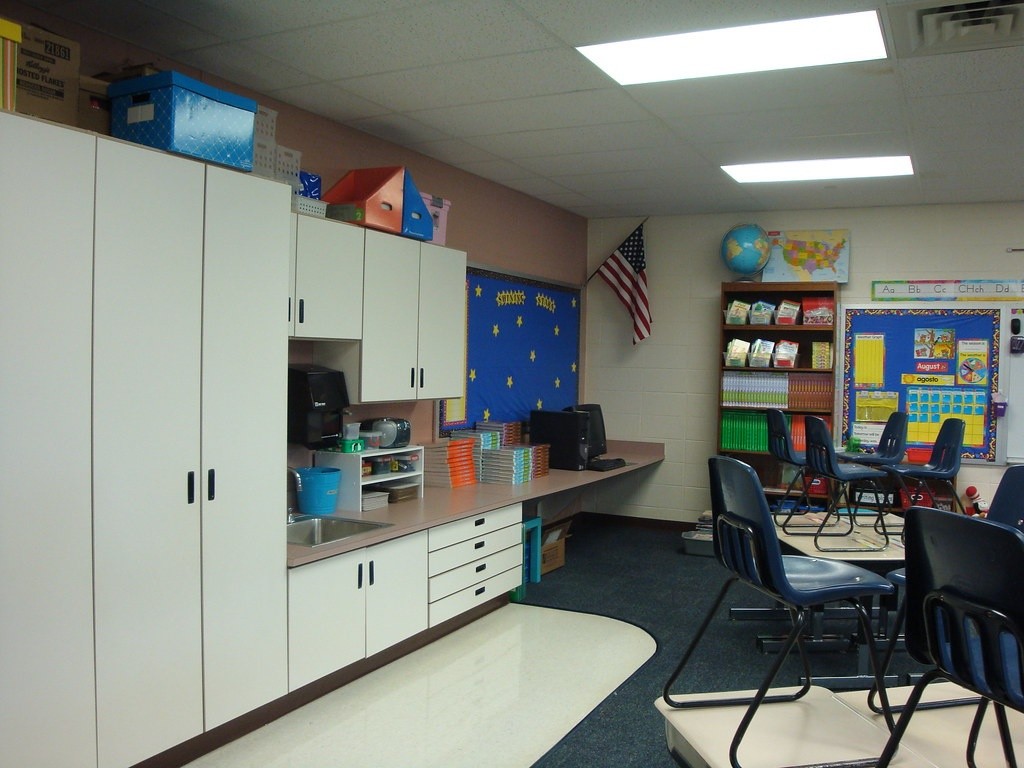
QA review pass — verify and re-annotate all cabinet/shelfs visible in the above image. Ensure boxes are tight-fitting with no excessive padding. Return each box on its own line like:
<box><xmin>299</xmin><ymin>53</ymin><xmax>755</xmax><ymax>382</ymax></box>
<box><xmin>313</xmin><ymin>445</ymin><xmax>425</xmax><ymax>511</ymax></box>
<box><xmin>313</xmin><ymin>228</ymin><xmax>468</xmax><ymax>405</ymax></box>
<box><xmin>0</xmin><ymin>109</ymin><xmax>96</xmax><ymax>768</ymax></box>
<box><xmin>287</xmin><ymin>529</ymin><xmax>429</xmax><ymax>695</ymax></box>
<box><xmin>91</xmin><ymin>130</ymin><xmax>288</xmax><ymax>768</ymax></box>
<box><xmin>714</xmin><ymin>282</ymin><xmax>841</xmax><ymax>507</ymax></box>
<box><xmin>287</xmin><ymin>212</ymin><xmax>365</xmax><ymax>341</ymax></box>
<box><xmin>427</xmin><ymin>502</ymin><xmax>523</xmax><ymax>629</ymax></box>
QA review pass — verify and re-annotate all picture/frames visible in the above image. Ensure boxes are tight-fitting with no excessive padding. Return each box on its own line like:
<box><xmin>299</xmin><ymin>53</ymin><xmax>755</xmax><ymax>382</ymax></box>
<box><xmin>432</xmin><ymin>262</ymin><xmax>588</xmax><ymax>442</ymax></box>
<box><xmin>836</xmin><ymin>308</ymin><xmax>1001</xmax><ymax>463</ymax></box>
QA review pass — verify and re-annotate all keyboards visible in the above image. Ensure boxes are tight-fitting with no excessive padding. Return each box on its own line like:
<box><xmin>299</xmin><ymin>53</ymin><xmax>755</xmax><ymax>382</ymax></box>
<box><xmin>587</xmin><ymin>459</ymin><xmax>626</xmax><ymax>471</ymax></box>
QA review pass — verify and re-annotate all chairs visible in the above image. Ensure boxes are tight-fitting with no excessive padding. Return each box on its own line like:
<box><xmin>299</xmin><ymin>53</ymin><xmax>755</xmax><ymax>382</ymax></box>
<box><xmin>766</xmin><ymin>408</ymin><xmax>841</xmax><ymax>527</ymax></box>
<box><xmin>879</xmin><ymin>505</ymin><xmax>1024</xmax><ymax>768</ymax></box>
<box><xmin>663</xmin><ymin>454</ymin><xmax>895</xmax><ymax>768</ymax></box>
<box><xmin>833</xmin><ymin>413</ymin><xmax>909</xmax><ymax>515</ymax></box>
<box><xmin>783</xmin><ymin>416</ymin><xmax>889</xmax><ymax>552</ymax></box>
<box><xmin>869</xmin><ymin>466</ymin><xmax>1024</xmax><ymax>714</ymax></box>
<box><xmin>874</xmin><ymin>418</ymin><xmax>966</xmax><ymax>535</ymax></box>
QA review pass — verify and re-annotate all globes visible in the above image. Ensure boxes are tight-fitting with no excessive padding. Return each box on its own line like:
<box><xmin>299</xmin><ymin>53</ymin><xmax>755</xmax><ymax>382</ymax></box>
<box><xmin>719</xmin><ymin>223</ymin><xmax>771</xmax><ymax>283</ymax></box>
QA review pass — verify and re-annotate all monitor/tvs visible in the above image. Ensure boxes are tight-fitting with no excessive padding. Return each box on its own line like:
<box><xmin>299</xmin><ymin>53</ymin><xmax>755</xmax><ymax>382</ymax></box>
<box><xmin>562</xmin><ymin>404</ymin><xmax>607</xmax><ymax>463</ymax></box>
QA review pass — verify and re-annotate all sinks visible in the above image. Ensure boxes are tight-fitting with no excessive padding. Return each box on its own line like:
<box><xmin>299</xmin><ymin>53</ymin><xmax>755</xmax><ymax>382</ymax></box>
<box><xmin>287</xmin><ymin>514</ymin><xmax>397</xmax><ymax>548</ymax></box>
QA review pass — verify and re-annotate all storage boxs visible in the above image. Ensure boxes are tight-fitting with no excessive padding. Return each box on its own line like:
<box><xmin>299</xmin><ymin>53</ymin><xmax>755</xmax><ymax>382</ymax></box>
<box><xmin>294</xmin><ymin>171</ymin><xmax>321</xmax><ymax>200</ymax></box>
<box><xmin>379</xmin><ymin>480</ymin><xmax>420</xmax><ymax>503</ymax></box>
<box><xmin>933</xmin><ymin>494</ymin><xmax>951</xmax><ymax>511</ymax></box>
<box><xmin>721</xmin><ymin>309</ymin><xmax>800</xmax><ymax>326</ymax></box>
<box><xmin>541</xmin><ymin>519</ymin><xmax>574</xmax><ymax>576</ymax></box>
<box><xmin>255</xmin><ymin>104</ymin><xmax>277</xmax><ymax>140</ymax></box>
<box><xmin>681</xmin><ymin>531</ymin><xmax>715</xmax><ymax>556</ymax></box>
<box><xmin>722</xmin><ymin>350</ymin><xmax>800</xmax><ymax>369</ymax></box>
<box><xmin>276</xmin><ymin>144</ymin><xmax>303</xmax><ymax>186</ymax></box>
<box><xmin>291</xmin><ymin>195</ymin><xmax>329</xmax><ymax>217</ymax></box>
<box><xmin>798</xmin><ymin>506</ymin><xmax>823</xmax><ymax>512</ymax></box>
<box><xmin>78</xmin><ymin>75</ymin><xmax>112</xmax><ymax>132</ymax></box>
<box><xmin>418</xmin><ymin>192</ymin><xmax>451</xmax><ymax>246</ymax></box>
<box><xmin>855</xmin><ymin>486</ymin><xmax>895</xmax><ymax>508</ymax></box>
<box><xmin>362</xmin><ymin>490</ymin><xmax>390</xmax><ymax>512</ymax></box>
<box><xmin>251</xmin><ymin>136</ymin><xmax>275</xmax><ymax>177</ymax></box>
<box><xmin>899</xmin><ymin>485</ymin><xmax>935</xmax><ymax>510</ymax></box>
<box><xmin>106</xmin><ymin>70</ymin><xmax>259</xmax><ymax>172</ymax></box>
<box><xmin>401</xmin><ymin>167</ymin><xmax>433</xmax><ymax>241</ymax></box>
<box><xmin>14</xmin><ymin>19</ymin><xmax>82</xmax><ymax>129</ymax></box>
<box><xmin>777</xmin><ymin>498</ymin><xmax>796</xmax><ymax>509</ymax></box>
<box><xmin>803</xmin><ymin>477</ymin><xmax>827</xmax><ymax>494</ymax></box>
<box><xmin>0</xmin><ymin>18</ymin><xmax>22</xmax><ymax>112</ymax></box>
<box><xmin>767</xmin><ymin>463</ymin><xmax>804</xmax><ymax>489</ymax></box>
<box><xmin>365</xmin><ymin>457</ymin><xmax>391</xmax><ymax>475</ymax></box>
<box><xmin>323</xmin><ymin>167</ymin><xmax>405</xmax><ymax>233</ymax></box>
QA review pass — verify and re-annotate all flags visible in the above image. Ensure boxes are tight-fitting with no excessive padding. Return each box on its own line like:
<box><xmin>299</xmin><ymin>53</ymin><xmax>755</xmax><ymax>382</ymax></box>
<box><xmin>595</xmin><ymin>223</ymin><xmax>653</xmax><ymax>346</ymax></box>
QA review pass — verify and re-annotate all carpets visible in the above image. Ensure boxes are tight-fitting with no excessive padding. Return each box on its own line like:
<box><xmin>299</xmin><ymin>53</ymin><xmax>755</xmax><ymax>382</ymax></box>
<box><xmin>511</xmin><ymin>513</ymin><xmax>949</xmax><ymax>768</ymax></box>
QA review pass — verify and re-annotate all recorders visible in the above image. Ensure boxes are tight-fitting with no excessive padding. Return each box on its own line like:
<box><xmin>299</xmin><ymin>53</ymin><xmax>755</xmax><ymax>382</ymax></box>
<box><xmin>358</xmin><ymin>417</ymin><xmax>410</xmax><ymax>449</ymax></box>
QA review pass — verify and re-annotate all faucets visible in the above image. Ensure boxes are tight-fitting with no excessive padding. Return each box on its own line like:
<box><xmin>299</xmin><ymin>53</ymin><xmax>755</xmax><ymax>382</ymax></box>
<box><xmin>287</xmin><ymin>467</ymin><xmax>302</xmax><ymax>492</ymax></box>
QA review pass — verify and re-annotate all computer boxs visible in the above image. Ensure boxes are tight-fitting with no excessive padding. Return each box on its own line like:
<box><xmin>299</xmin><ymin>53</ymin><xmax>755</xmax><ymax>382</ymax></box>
<box><xmin>529</xmin><ymin>409</ymin><xmax>590</xmax><ymax>471</ymax></box>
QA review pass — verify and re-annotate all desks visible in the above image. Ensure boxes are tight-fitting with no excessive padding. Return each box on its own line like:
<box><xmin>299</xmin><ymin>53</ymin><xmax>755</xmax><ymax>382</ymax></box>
<box><xmin>290</xmin><ymin>436</ymin><xmax>664</xmax><ymax>569</ymax></box>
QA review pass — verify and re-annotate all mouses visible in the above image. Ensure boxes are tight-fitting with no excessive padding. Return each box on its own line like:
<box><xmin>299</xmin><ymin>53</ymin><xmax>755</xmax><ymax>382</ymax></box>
<box><xmin>615</xmin><ymin>458</ymin><xmax>625</xmax><ymax>463</ymax></box>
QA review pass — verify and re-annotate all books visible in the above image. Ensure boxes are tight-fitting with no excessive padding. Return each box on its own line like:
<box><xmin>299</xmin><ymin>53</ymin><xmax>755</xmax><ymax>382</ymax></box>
<box><xmin>726</xmin><ymin>338</ymin><xmax>834</xmax><ymax>369</ymax></box>
<box><xmin>721</xmin><ymin>371</ymin><xmax>834</xmax><ymax>409</ymax></box>
<box><xmin>721</xmin><ymin>412</ymin><xmax>831</xmax><ymax>451</ymax></box>
<box><xmin>417</xmin><ymin>420</ymin><xmax>551</xmax><ymax>489</ymax></box>
<box><xmin>725</xmin><ymin>297</ymin><xmax>834</xmax><ymax>326</ymax></box>
